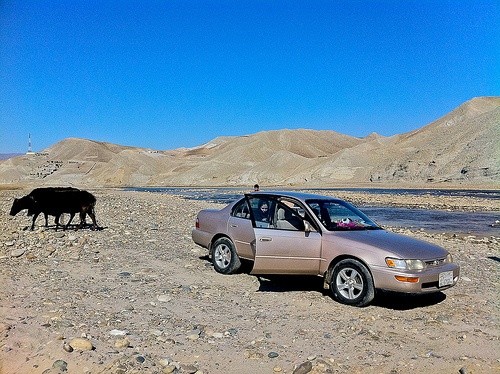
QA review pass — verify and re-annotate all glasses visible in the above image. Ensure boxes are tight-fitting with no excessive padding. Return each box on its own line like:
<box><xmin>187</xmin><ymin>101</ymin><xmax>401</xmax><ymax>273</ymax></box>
<box><xmin>254</xmin><ymin>190</ymin><xmax>259</xmax><ymax>191</ymax></box>
<box><xmin>261</xmin><ymin>208</ymin><xmax>268</xmax><ymax>209</ymax></box>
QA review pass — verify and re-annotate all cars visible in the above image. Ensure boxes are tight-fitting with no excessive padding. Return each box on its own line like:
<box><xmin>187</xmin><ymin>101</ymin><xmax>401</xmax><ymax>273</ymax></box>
<box><xmin>192</xmin><ymin>190</ymin><xmax>460</xmax><ymax>308</ymax></box>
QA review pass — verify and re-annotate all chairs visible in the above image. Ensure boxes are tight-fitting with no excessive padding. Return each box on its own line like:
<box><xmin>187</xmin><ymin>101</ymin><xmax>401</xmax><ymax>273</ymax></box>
<box><xmin>277</xmin><ymin>210</ymin><xmax>318</xmax><ymax>232</ymax></box>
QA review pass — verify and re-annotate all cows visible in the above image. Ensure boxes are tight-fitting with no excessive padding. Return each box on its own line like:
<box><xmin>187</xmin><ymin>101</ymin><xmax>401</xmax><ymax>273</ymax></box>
<box><xmin>26</xmin><ymin>190</ymin><xmax>99</xmax><ymax>231</ymax></box>
<box><xmin>8</xmin><ymin>186</ymin><xmax>80</xmax><ymax>231</ymax></box>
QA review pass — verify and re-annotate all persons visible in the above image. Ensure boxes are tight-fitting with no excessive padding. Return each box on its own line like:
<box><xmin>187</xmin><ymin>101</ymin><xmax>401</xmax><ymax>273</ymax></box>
<box><xmin>250</xmin><ymin>199</ymin><xmax>271</xmax><ymax>222</ymax></box>
<box><xmin>302</xmin><ymin>208</ymin><xmax>327</xmax><ymax>233</ymax></box>
<box><xmin>247</xmin><ymin>184</ymin><xmax>261</xmax><ymax>209</ymax></box>
